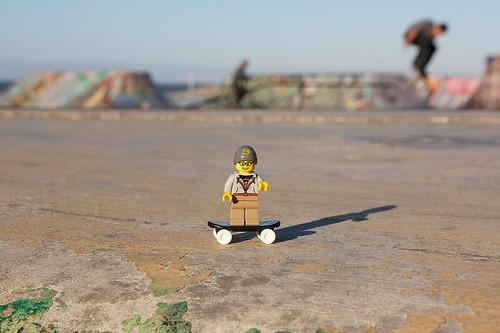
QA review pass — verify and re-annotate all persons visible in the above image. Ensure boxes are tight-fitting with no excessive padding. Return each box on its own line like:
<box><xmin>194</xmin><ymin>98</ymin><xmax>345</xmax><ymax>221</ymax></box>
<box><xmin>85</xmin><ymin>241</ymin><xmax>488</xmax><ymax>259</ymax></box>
<box><xmin>222</xmin><ymin>145</ymin><xmax>269</xmax><ymax>225</ymax></box>
<box><xmin>230</xmin><ymin>59</ymin><xmax>250</xmax><ymax>105</ymax></box>
<box><xmin>405</xmin><ymin>20</ymin><xmax>448</xmax><ymax>79</ymax></box>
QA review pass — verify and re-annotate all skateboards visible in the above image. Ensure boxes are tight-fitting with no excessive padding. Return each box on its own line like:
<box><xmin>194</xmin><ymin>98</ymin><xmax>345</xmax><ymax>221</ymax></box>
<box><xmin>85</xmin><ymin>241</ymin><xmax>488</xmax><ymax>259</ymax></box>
<box><xmin>208</xmin><ymin>218</ymin><xmax>280</xmax><ymax>245</ymax></box>
